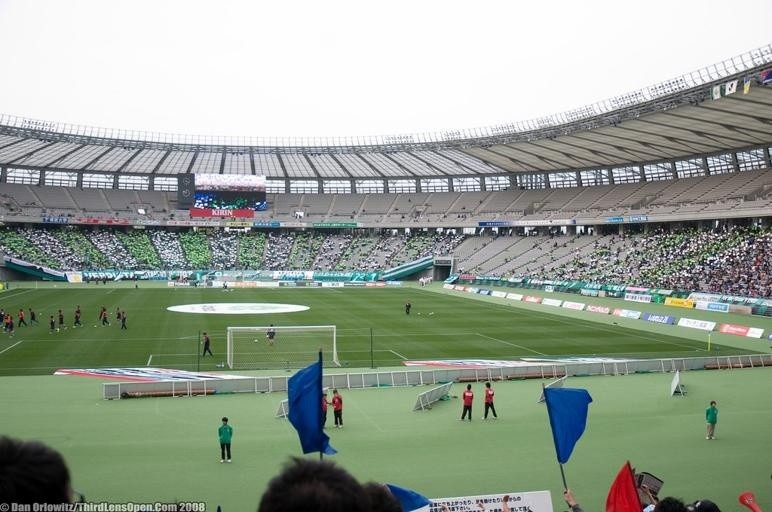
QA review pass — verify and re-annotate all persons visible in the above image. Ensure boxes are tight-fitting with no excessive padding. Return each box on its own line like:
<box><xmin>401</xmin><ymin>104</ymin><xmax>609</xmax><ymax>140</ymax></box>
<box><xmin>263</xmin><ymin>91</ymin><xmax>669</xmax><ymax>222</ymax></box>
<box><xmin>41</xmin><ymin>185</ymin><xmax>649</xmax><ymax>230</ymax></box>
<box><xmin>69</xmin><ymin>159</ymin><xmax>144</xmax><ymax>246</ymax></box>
<box><xmin>0</xmin><ymin>223</ymin><xmax>772</xmax><ymax>339</ymax></box>
<box><xmin>266</xmin><ymin>324</ymin><xmax>276</xmax><ymax>346</ymax></box>
<box><xmin>481</xmin><ymin>380</ymin><xmax>497</xmax><ymax>421</ymax></box>
<box><xmin>198</xmin><ymin>331</ymin><xmax>213</xmax><ymax>361</ymax></box>
<box><xmin>705</xmin><ymin>400</ymin><xmax>718</xmax><ymax>441</ymax></box>
<box><xmin>331</xmin><ymin>388</ymin><xmax>345</xmax><ymax>428</ymax></box>
<box><xmin>217</xmin><ymin>416</ymin><xmax>233</xmax><ymax>463</ymax></box>
<box><xmin>0</xmin><ymin>435</ymin><xmax>737</xmax><ymax>511</ymax></box>
<box><xmin>320</xmin><ymin>393</ymin><xmax>330</xmax><ymax>428</ymax></box>
<box><xmin>458</xmin><ymin>384</ymin><xmax>475</xmax><ymax>421</ymax></box>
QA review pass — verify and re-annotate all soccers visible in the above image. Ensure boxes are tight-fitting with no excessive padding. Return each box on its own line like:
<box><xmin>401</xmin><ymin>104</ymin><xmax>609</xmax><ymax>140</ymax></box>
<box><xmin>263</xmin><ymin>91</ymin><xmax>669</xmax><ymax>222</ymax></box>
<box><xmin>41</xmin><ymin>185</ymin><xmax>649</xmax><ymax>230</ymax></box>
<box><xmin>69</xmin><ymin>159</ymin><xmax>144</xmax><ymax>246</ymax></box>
<box><xmin>39</xmin><ymin>312</ymin><xmax>44</xmax><ymax>316</ymax></box>
<box><xmin>416</xmin><ymin>311</ymin><xmax>434</xmax><ymax>317</ymax></box>
<box><xmin>254</xmin><ymin>338</ymin><xmax>259</xmax><ymax>342</ymax></box>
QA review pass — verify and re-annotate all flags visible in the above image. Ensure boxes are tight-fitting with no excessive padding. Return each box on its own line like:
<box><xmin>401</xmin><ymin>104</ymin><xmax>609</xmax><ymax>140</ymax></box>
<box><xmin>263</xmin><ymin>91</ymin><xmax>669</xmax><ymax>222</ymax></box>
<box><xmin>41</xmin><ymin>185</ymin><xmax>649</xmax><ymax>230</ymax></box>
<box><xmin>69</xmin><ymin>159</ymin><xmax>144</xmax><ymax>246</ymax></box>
<box><xmin>284</xmin><ymin>358</ymin><xmax>336</xmax><ymax>455</ymax></box>
<box><xmin>539</xmin><ymin>385</ymin><xmax>592</xmax><ymax>462</ymax></box>
<box><xmin>707</xmin><ymin>68</ymin><xmax>772</xmax><ymax>101</ymax></box>
